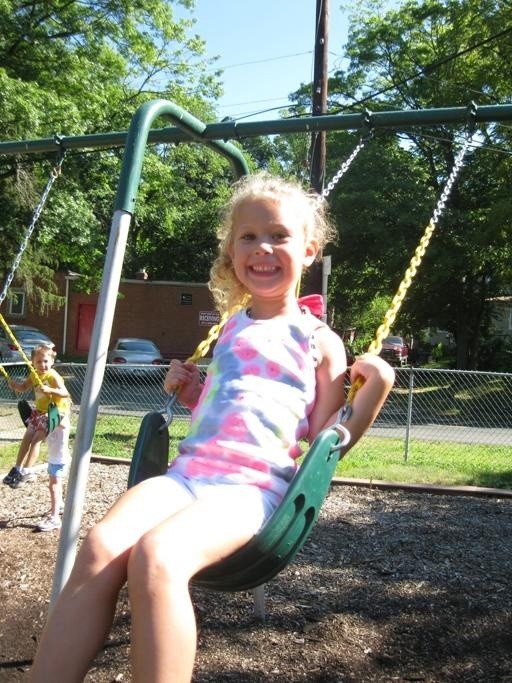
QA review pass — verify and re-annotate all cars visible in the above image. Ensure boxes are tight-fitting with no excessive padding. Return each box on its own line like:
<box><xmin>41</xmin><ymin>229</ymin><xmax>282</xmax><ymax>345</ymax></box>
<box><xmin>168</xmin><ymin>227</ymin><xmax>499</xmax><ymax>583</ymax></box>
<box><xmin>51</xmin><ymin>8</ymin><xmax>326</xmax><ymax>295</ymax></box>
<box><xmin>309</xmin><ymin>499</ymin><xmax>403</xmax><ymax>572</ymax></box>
<box><xmin>343</xmin><ymin>342</ymin><xmax>357</xmax><ymax>367</ymax></box>
<box><xmin>108</xmin><ymin>336</ymin><xmax>166</xmax><ymax>382</ymax></box>
<box><xmin>0</xmin><ymin>320</ymin><xmax>56</xmax><ymax>366</ymax></box>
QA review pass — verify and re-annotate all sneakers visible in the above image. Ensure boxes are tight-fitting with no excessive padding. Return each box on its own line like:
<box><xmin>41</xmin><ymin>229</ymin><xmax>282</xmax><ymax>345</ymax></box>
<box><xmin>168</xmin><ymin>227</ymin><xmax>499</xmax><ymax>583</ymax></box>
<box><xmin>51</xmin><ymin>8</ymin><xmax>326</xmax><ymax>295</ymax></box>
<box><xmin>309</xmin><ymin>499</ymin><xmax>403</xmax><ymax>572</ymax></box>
<box><xmin>4</xmin><ymin>469</ymin><xmax>37</xmax><ymax>488</ymax></box>
<box><xmin>38</xmin><ymin>502</ymin><xmax>66</xmax><ymax>531</ymax></box>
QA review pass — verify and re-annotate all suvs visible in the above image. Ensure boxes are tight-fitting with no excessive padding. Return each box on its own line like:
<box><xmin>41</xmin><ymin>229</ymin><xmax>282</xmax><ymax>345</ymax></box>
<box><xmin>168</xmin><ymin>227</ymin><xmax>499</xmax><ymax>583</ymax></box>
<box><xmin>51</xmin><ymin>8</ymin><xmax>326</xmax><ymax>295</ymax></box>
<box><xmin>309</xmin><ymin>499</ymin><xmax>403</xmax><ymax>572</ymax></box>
<box><xmin>378</xmin><ymin>335</ymin><xmax>410</xmax><ymax>367</ymax></box>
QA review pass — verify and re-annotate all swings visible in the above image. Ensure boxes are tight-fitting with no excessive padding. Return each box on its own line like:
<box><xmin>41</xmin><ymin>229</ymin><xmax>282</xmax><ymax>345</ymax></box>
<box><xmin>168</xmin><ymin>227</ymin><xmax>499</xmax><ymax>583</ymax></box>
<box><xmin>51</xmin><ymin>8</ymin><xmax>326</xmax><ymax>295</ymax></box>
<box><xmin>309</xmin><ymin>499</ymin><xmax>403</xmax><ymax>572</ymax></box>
<box><xmin>0</xmin><ymin>151</ymin><xmax>65</xmax><ymax>435</ymax></box>
<box><xmin>127</xmin><ymin>122</ymin><xmax>475</xmax><ymax>589</ymax></box>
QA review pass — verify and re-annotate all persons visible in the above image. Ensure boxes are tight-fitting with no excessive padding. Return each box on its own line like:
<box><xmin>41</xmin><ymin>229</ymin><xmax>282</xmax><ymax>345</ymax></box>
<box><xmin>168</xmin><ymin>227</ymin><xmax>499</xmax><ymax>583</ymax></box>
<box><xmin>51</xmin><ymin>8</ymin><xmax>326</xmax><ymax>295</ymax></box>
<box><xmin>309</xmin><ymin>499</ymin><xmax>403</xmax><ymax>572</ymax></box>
<box><xmin>28</xmin><ymin>170</ymin><xmax>396</xmax><ymax>683</ymax></box>
<box><xmin>3</xmin><ymin>347</ymin><xmax>72</xmax><ymax>532</ymax></box>
